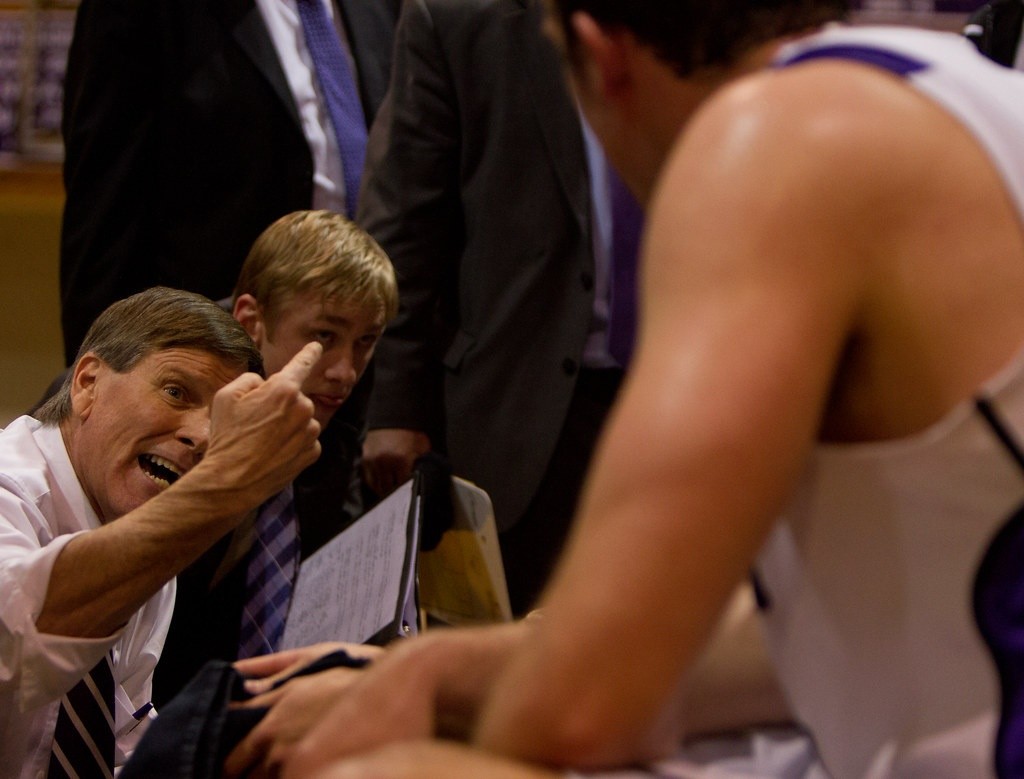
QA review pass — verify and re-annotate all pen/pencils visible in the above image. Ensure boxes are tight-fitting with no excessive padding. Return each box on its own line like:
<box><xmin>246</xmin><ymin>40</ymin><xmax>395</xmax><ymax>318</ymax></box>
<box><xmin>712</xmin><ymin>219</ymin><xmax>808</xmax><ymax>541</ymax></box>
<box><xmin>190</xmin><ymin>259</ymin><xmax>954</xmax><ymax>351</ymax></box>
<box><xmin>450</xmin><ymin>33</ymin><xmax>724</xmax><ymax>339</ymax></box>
<box><xmin>116</xmin><ymin>701</ymin><xmax>154</xmax><ymax>741</ymax></box>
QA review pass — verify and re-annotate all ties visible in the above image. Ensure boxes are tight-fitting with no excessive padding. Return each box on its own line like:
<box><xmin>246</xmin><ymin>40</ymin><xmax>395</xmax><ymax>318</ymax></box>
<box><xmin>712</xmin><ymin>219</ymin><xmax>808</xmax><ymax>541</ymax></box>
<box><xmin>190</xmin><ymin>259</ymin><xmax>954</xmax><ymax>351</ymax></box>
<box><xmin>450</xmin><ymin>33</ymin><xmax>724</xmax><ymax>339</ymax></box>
<box><xmin>292</xmin><ymin>0</ymin><xmax>369</xmax><ymax>227</ymax></box>
<box><xmin>45</xmin><ymin>641</ymin><xmax>119</xmax><ymax>778</ymax></box>
<box><xmin>602</xmin><ymin>153</ymin><xmax>645</xmax><ymax>361</ymax></box>
<box><xmin>240</xmin><ymin>477</ymin><xmax>300</xmax><ymax>663</ymax></box>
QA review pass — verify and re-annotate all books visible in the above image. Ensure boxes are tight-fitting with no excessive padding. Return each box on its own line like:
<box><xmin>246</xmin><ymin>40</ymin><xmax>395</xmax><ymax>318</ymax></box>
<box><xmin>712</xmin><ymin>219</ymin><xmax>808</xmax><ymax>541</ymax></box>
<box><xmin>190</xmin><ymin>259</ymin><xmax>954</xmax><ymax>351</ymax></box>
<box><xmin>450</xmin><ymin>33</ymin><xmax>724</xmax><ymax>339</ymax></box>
<box><xmin>276</xmin><ymin>475</ymin><xmax>513</xmax><ymax>651</ymax></box>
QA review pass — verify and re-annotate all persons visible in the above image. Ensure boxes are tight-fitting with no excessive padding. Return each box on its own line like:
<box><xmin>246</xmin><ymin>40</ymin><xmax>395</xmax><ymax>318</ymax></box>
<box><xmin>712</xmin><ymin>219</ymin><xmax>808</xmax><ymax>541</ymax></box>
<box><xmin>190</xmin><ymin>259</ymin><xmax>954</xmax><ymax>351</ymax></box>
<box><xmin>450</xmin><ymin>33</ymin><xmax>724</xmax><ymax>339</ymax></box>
<box><xmin>59</xmin><ymin>1</ymin><xmax>400</xmax><ymax>372</ymax></box>
<box><xmin>225</xmin><ymin>0</ymin><xmax>1024</xmax><ymax>779</ymax></box>
<box><xmin>0</xmin><ymin>210</ymin><xmax>397</xmax><ymax>779</ymax></box>
